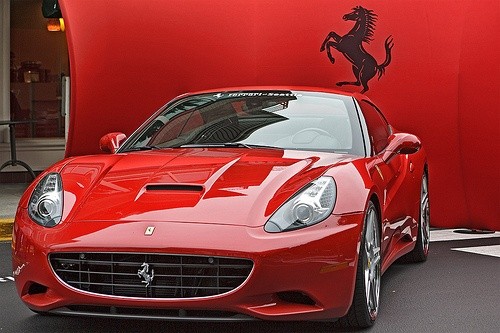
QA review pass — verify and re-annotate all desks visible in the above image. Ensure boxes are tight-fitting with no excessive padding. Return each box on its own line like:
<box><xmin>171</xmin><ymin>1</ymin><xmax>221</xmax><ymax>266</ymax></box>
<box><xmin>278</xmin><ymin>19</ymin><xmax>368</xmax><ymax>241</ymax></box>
<box><xmin>0</xmin><ymin>120</ymin><xmax>37</xmax><ymax>180</ymax></box>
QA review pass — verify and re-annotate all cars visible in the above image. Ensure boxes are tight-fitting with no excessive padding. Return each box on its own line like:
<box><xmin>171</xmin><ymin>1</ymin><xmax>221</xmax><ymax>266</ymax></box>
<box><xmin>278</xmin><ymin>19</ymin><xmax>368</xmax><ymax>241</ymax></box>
<box><xmin>11</xmin><ymin>86</ymin><xmax>430</xmax><ymax>333</ymax></box>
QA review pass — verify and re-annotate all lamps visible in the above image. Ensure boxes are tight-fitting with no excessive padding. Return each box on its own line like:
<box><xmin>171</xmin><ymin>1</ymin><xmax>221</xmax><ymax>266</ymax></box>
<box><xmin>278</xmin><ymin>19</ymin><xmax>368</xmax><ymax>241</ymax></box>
<box><xmin>42</xmin><ymin>0</ymin><xmax>64</xmax><ymax>31</ymax></box>
